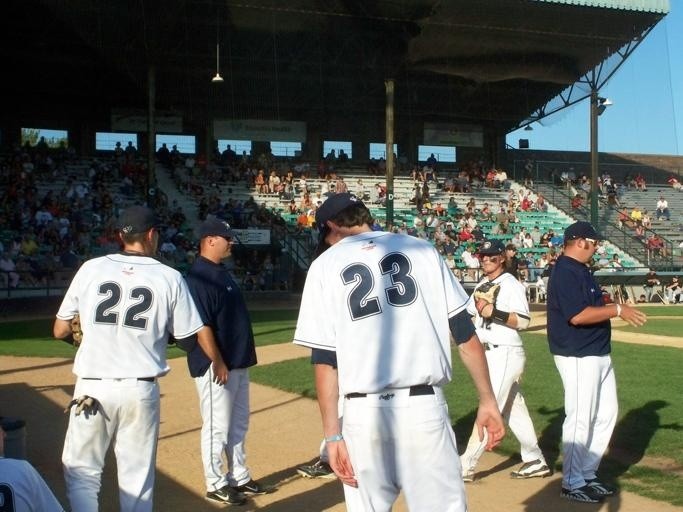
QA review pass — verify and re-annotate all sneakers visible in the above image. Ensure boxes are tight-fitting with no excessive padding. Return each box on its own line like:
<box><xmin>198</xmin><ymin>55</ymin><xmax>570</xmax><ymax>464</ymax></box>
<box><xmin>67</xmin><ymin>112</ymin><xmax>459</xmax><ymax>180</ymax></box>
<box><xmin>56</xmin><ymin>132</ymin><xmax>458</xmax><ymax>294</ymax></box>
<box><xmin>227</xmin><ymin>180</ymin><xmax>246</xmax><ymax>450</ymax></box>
<box><xmin>561</xmin><ymin>485</ymin><xmax>602</xmax><ymax>503</ymax></box>
<box><xmin>232</xmin><ymin>479</ymin><xmax>267</xmax><ymax>494</ymax></box>
<box><xmin>510</xmin><ymin>459</ymin><xmax>552</xmax><ymax>478</ymax></box>
<box><xmin>206</xmin><ymin>485</ymin><xmax>246</xmax><ymax>506</ymax></box>
<box><xmin>296</xmin><ymin>460</ymin><xmax>337</xmax><ymax>479</ymax></box>
<box><xmin>584</xmin><ymin>477</ymin><xmax>617</xmax><ymax>497</ymax></box>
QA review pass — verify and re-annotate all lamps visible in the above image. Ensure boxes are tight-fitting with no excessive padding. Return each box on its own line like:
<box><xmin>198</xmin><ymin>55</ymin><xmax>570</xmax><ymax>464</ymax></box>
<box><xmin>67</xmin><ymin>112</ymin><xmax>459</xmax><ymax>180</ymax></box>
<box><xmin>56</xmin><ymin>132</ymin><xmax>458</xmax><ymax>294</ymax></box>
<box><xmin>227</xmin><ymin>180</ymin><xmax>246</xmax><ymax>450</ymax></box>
<box><xmin>211</xmin><ymin>0</ymin><xmax>224</xmax><ymax>82</ymax></box>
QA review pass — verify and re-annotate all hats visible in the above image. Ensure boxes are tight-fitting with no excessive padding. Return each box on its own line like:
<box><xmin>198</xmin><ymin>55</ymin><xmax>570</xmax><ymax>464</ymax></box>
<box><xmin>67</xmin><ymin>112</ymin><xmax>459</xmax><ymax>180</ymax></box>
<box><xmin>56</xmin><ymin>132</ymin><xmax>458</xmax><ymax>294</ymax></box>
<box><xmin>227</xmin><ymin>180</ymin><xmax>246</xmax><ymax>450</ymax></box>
<box><xmin>199</xmin><ymin>218</ymin><xmax>238</xmax><ymax>238</ymax></box>
<box><xmin>116</xmin><ymin>205</ymin><xmax>161</xmax><ymax>233</ymax></box>
<box><xmin>315</xmin><ymin>193</ymin><xmax>364</xmax><ymax>254</ymax></box>
<box><xmin>478</xmin><ymin>240</ymin><xmax>505</xmax><ymax>255</ymax></box>
<box><xmin>1</xmin><ymin>414</ymin><xmax>26</xmax><ymax>431</ymax></box>
<box><xmin>564</xmin><ymin>221</ymin><xmax>606</xmax><ymax>241</ymax></box>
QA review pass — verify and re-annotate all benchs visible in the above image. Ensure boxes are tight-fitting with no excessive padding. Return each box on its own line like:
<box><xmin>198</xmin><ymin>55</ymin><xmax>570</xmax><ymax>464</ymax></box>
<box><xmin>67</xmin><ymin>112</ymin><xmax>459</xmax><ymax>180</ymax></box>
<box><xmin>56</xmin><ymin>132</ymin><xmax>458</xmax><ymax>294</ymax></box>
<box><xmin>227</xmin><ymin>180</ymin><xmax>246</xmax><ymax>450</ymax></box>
<box><xmin>0</xmin><ymin>146</ymin><xmax>170</xmax><ymax>300</ymax></box>
<box><xmin>519</xmin><ymin>178</ymin><xmax>683</xmax><ymax>270</ymax></box>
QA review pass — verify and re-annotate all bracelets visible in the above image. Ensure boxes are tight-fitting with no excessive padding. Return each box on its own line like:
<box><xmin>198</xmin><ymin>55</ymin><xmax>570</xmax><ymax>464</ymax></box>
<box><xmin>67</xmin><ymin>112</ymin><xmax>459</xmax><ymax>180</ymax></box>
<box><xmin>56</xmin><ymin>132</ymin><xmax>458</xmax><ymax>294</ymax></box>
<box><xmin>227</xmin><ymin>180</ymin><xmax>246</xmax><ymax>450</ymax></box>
<box><xmin>486</xmin><ymin>308</ymin><xmax>510</xmax><ymax>325</ymax></box>
<box><xmin>617</xmin><ymin>303</ymin><xmax>622</xmax><ymax>316</ymax></box>
<box><xmin>322</xmin><ymin>433</ymin><xmax>343</xmax><ymax>443</ymax></box>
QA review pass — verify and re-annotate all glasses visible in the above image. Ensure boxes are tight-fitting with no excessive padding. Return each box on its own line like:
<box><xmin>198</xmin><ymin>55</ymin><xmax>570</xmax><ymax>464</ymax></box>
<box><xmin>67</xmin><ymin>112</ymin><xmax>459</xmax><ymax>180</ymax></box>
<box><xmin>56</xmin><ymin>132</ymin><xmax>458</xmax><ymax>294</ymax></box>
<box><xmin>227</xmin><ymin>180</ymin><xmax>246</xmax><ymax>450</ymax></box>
<box><xmin>582</xmin><ymin>237</ymin><xmax>598</xmax><ymax>247</ymax></box>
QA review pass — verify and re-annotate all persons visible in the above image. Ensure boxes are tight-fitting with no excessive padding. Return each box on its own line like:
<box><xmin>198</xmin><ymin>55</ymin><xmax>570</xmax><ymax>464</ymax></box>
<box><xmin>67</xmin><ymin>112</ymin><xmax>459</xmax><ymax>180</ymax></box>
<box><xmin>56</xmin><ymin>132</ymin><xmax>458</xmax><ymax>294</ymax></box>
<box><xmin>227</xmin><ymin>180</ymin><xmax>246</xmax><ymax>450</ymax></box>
<box><xmin>179</xmin><ymin>219</ymin><xmax>269</xmax><ymax>506</ymax></box>
<box><xmin>460</xmin><ymin>239</ymin><xmax>551</xmax><ymax>483</ymax></box>
<box><xmin>545</xmin><ymin>221</ymin><xmax>648</xmax><ymax>504</ymax></box>
<box><xmin>292</xmin><ymin>193</ymin><xmax>507</xmax><ymax>512</ymax></box>
<box><xmin>296</xmin><ymin>387</ymin><xmax>344</xmax><ymax>480</ymax></box>
<box><xmin>153</xmin><ymin>140</ymin><xmax>387</xmax><ymax>293</ymax></box>
<box><xmin>394</xmin><ymin>150</ymin><xmax>683</xmax><ymax>305</ymax></box>
<box><xmin>1</xmin><ymin>135</ymin><xmax>149</xmax><ymax>290</ymax></box>
<box><xmin>52</xmin><ymin>206</ymin><xmax>230</xmax><ymax>511</ymax></box>
<box><xmin>0</xmin><ymin>421</ymin><xmax>64</xmax><ymax>512</ymax></box>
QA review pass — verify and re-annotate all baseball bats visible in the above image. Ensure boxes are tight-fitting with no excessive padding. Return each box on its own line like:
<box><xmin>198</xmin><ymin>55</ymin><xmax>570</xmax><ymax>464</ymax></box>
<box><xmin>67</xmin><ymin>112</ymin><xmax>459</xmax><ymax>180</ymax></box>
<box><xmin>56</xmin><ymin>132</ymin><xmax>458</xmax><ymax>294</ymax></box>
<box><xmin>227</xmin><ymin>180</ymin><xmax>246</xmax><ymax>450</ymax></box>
<box><xmin>611</xmin><ymin>284</ymin><xmax>634</xmax><ymax>304</ymax></box>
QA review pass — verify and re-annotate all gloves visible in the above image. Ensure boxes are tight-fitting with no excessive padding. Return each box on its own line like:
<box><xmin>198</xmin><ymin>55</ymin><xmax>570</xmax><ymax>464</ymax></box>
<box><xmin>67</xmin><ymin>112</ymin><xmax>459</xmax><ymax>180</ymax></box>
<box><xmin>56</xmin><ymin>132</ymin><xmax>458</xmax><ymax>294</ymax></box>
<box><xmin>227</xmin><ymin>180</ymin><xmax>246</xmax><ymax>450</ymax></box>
<box><xmin>64</xmin><ymin>395</ymin><xmax>95</xmax><ymax>415</ymax></box>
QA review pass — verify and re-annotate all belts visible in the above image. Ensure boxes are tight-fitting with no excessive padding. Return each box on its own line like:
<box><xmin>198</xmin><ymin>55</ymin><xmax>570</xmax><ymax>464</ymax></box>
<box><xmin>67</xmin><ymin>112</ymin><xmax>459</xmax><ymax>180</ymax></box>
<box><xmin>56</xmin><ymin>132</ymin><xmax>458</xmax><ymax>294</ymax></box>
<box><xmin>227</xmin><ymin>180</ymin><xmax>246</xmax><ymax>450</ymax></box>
<box><xmin>345</xmin><ymin>384</ymin><xmax>434</xmax><ymax>400</ymax></box>
<box><xmin>481</xmin><ymin>344</ymin><xmax>498</xmax><ymax>350</ymax></box>
<box><xmin>82</xmin><ymin>377</ymin><xmax>154</xmax><ymax>382</ymax></box>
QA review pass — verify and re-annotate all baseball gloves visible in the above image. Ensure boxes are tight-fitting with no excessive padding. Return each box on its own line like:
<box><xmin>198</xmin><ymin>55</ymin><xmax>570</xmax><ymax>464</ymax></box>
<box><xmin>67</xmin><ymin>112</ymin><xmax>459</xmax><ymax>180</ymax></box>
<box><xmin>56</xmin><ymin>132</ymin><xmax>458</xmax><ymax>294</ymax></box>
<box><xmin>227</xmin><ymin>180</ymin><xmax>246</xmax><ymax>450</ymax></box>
<box><xmin>71</xmin><ymin>314</ymin><xmax>82</xmax><ymax>346</ymax></box>
<box><xmin>474</xmin><ymin>282</ymin><xmax>501</xmax><ymax>306</ymax></box>
